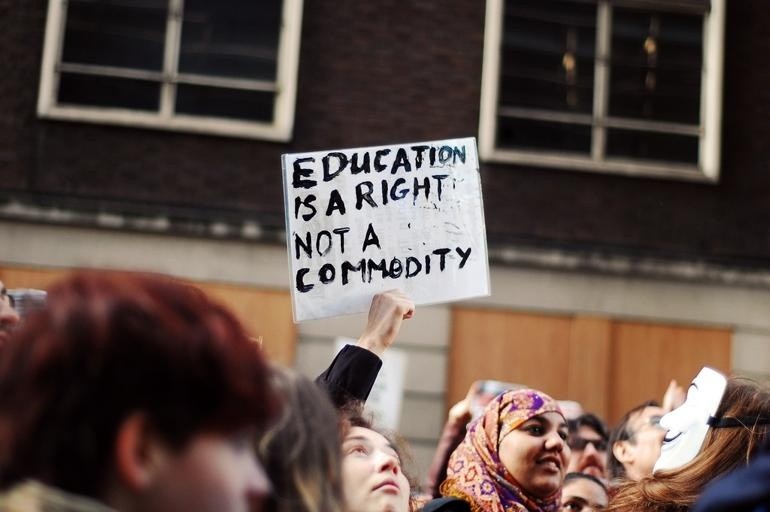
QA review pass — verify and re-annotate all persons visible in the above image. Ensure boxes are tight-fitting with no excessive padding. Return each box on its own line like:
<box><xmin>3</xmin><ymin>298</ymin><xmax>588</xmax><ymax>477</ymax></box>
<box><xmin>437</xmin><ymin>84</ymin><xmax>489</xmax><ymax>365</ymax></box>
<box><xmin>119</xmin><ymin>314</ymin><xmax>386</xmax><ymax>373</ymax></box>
<box><xmin>412</xmin><ymin>367</ymin><xmax>770</xmax><ymax>512</ymax></box>
<box><xmin>0</xmin><ymin>266</ymin><xmax>411</xmax><ymax>512</ymax></box>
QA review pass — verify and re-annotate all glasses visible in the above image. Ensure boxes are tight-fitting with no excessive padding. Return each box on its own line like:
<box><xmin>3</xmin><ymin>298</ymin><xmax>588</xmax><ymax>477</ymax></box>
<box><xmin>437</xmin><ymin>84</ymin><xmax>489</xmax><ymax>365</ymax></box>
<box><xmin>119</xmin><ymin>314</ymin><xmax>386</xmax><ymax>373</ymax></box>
<box><xmin>566</xmin><ymin>437</ymin><xmax>605</xmax><ymax>451</ymax></box>
